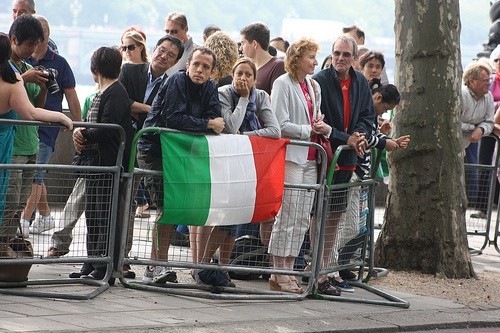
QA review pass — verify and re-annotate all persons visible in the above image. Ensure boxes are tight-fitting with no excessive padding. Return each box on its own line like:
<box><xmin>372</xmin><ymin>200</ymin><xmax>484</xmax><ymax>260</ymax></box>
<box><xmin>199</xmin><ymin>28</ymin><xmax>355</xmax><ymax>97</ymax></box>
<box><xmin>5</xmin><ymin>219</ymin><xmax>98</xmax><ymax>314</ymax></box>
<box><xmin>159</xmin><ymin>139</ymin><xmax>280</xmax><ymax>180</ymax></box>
<box><xmin>190</xmin><ymin>57</ymin><xmax>282</xmax><ymax>287</ymax></box>
<box><xmin>267</xmin><ymin>39</ymin><xmax>332</xmax><ymax>294</ymax></box>
<box><xmin>12</xmin><ymin>0</ymin><xmax>59</xmax><ymax>54</ymax></box>
<box><xmin>271</xmin><ymin>37</ymin><xmax>290</xmax><ymax>53</ymax></box>
<box><xmin>310</xmin><ymin>26</ymin><xmax>410</xmax><ymax>296</ymax></box>
<box><xmin>0</xmin><ymin>32</ymin><xmax>73</xmax><ymax>226</ymax></box>
<box><xmin>470</xmin><ymin>44</ymin><xmax>500</xmax><ymax>219</ymax></box>
<box><xmin>43</xmin><ymin>91</ymin><xmax>100</xmax><ymax>257</ymax></box>
<box><xmin>17</xmin><ymin>14</ymin><xmax>82</xmax><ymax>237</ymax></box>
<box><xmin>120</xmin><ymin>26</ymin><xmax>148</xmax><ymax>64</ymax></box>
<box><xmin>137</xmin><ymin>47</ymin><xmax>225</xmax><ymax>287</ymax></box>
<box><xmin>72</xmin><ymin>47</ymin><xmax>134</xmax><ymax>281</ymax></box>
<box><xmin>165</xmin><ymin>14</ymin><xmax>200</xmax><ymax>76</ymax></box>
<box><xmin>462</xmin><ymin>61</ymin><xmax>494</xmax><ymax>154</ymax></box>
<box><xmin>0</xmin><ymin>15</ymin><xmax>49</xmax><ymax>242</ymax></box>
<box><xmin>119</xmin><ymin>35</ymin><xmax>185</xmax><ymax>278</ymax></box>
<box><xmin>239</xmin><ymin>23</ymin><xmax>287</xmax><ymax>97</ymax></box>
<box><xmin>190</xmin><ymin>25</ymin><xmax>239</xmax><ymax>280</ymax></box>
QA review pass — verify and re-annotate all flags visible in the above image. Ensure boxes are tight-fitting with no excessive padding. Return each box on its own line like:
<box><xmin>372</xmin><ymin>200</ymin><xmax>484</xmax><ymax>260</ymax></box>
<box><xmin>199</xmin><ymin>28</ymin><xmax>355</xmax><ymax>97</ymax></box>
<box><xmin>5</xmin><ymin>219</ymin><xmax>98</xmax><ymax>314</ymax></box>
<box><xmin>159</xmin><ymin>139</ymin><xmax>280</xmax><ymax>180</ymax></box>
<box><xmin>158</xmin><ymin>130</ymin><xmax>286</xmax><ymax>226</ymax></box>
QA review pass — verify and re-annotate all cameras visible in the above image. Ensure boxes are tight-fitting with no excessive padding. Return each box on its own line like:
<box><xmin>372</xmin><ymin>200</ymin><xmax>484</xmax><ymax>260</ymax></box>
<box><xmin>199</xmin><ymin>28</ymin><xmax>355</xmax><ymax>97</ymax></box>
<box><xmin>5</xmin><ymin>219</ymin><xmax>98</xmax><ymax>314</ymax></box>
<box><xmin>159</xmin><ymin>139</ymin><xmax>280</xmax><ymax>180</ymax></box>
<box><xmin>42</xmin><ymin>68</ymin><xmax>60</xmax><ymax>95</ymax></box>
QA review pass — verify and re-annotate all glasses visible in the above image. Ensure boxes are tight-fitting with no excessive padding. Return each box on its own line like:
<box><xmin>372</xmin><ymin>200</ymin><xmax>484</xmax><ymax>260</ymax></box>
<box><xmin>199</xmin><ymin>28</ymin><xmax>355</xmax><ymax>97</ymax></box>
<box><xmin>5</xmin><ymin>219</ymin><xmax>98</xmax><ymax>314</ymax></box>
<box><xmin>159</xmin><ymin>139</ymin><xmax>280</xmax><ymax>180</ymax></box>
<box><xmin>494</xmin><ymin>58</ymin><xmax>500</xmax><ymax>62</ymax></box>
<box><xmin>332</xmin><ymin>50</ymin><xmax>354</xmax><ymax>58</ymax></box>
<box><xmin>121</xmin><ymin>44</ymin><xmax>138</xmax><ymax>52</ymax></box>
<box><xmin>164</xmin><ymin>29</ymin><xmax>182</xmax><ymax>35</ymax></box>
<box><xmin>382</xmin><ymin>104</ymin><xmax>389</xmax><ymax>112</ymax></box>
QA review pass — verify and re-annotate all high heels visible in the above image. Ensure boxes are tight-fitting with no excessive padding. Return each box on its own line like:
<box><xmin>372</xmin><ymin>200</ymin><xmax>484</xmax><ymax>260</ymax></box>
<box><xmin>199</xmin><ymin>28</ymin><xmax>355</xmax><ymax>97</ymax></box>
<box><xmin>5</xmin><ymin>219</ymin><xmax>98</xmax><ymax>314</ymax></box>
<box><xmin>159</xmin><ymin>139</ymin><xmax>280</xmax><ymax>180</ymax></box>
<box><xmin>269</xmin><ymin>274</ymin><xmax>304</xmax><ymax>293</ymax></box>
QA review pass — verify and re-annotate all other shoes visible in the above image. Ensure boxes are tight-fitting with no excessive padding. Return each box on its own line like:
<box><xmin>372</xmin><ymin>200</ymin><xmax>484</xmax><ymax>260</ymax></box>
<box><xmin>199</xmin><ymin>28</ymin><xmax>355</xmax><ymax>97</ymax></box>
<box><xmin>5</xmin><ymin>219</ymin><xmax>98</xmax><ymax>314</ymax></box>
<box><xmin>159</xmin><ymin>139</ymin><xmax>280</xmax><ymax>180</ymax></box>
<box><xmin>339</xmin><ymin>269</ymin><xmax>356</xmax><ymax>280</ymax></box>
<box><xmin>48</xmin><ymin>247</ymin><xmax>69</xmax><ymax>256</ymax></box>
<box><xmin>69</xmin><ymin>269</ymin><xmax>91</xmax><ymax>278</ymax></box>
<box><xmin>170</xmin><ymin>231</ymin><xmax>186</xmax><ymax>246</ymax></box>
<box><xmin>192</xmin><ymin>269</ymin><xmax>236</xmax><ymax>293</ymax></box>
<box><xmin>470</xmin><ymin>212</ymin><xmax>488</xmax><ymax>220</ymax></box>
<box><xmin>328</xmin><ymin>276</ymin><xmax>355</xmax><ymax>292</ymax></box>
<box><xmin>122</xmin><ymin>264</ymin><xmax>135</xmax><ymax>278</ymax></box>
<box><xmin>80</xmin><ymin>270</ymin><xmax>115</xmax><ymax>286</ymax></box>
<box><xmin>300</xmin><ymin>275</ymin><xmax>310</xmax><ymax>285</ymax></box>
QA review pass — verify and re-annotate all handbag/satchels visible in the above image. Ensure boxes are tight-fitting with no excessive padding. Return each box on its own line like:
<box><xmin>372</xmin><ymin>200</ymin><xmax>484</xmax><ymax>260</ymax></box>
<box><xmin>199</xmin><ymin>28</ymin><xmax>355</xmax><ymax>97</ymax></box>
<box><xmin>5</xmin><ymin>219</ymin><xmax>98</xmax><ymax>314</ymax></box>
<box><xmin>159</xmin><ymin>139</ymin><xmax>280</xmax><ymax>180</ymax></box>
<box><xmin>0</xmin><ymin>213</ymin><xmax>34</xmax><ymax>281</ymax></box>
<box><xmin>310</xmin><ymin>76</ymin><xmax>332</xmax><ymax>169</ymax></box>
<box><xmin>70</xmin><ymin>144</ymin><xmax>102</xmax><ymax>178</ymax></box>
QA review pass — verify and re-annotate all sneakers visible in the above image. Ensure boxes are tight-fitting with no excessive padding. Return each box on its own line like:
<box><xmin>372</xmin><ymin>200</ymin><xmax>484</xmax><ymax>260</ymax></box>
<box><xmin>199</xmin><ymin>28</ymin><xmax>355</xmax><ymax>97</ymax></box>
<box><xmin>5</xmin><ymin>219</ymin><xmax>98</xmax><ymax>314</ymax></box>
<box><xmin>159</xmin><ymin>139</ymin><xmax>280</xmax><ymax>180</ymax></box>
<box><xmin>153</xmin><ymin>266</ymin><xmax>177</xmax><ymax>283</ymax></box>
<box><xmin>16</xmin><ymin>222</ymin><xmax>29</xmax><ymax>238</ymax></box>
<box><xmin>29</xmin><ymin>215</ymin><xmax>55</xmax><ymax>233</ymax></box>
<box><xmin>312</xmin><ymin>280</ymin><xmax>341</xmax><ymax>296</ymax></box>
<box><xmin>142</xmin><ymin>265</ymin><xmax>178</xmax><ymax>287</ymax></box>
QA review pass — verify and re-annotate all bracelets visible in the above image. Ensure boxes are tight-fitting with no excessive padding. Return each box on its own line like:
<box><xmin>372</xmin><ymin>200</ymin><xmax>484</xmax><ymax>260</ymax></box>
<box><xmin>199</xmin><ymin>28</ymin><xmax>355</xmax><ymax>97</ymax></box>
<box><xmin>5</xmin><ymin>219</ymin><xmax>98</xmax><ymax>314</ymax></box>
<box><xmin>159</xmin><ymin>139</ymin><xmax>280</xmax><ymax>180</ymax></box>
<box><xmin>480</xmin><ymin>127</ymin><xmax>484</xmax><ymax>134</ymax></box>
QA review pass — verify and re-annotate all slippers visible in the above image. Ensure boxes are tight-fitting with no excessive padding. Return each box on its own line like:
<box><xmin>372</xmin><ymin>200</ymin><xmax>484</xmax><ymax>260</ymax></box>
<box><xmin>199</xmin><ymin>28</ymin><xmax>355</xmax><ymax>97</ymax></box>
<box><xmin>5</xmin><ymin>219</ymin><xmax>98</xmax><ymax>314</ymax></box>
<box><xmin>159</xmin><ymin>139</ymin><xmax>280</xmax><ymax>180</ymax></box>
<box><xmin>134</xmin><ymin>213</ymin><xmax>150</xmax><ymax>218</ymax></box>
